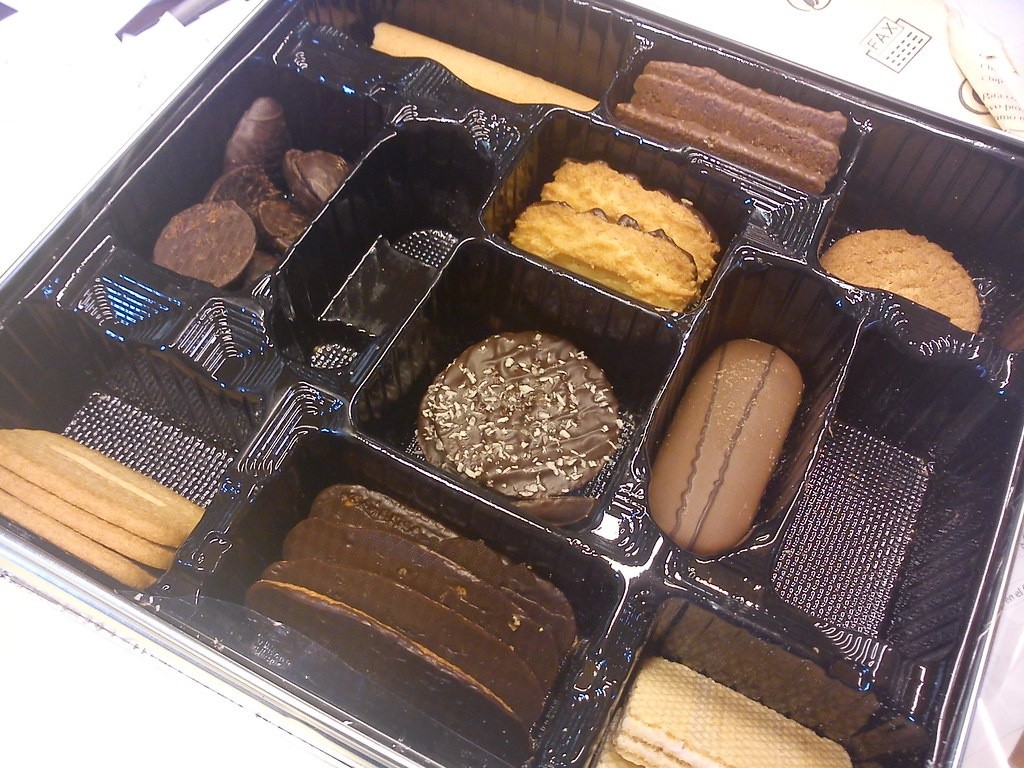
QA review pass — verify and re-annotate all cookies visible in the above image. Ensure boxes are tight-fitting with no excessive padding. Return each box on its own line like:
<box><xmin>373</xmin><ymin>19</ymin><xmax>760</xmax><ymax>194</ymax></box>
<box><xmin>0</xmin><ymin>19</ymin><xmax>983</xmax><ymax>768</ymax></box>
